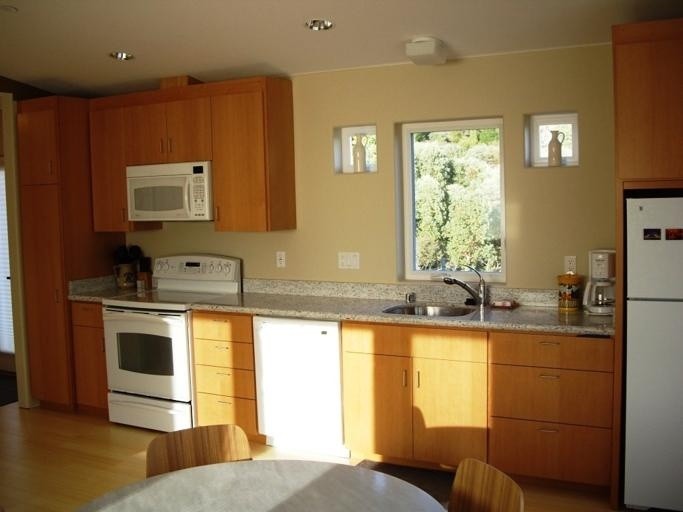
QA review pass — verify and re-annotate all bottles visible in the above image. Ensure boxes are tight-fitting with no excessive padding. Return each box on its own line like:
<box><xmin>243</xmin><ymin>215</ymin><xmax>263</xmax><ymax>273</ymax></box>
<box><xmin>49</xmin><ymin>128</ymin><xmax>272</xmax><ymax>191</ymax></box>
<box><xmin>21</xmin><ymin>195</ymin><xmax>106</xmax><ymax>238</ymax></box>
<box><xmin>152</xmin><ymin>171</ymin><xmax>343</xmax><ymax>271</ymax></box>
<box><xmin>136</xmin><ymin>280</ymin><xmax>146</xmax><ymax>289</ymax></box>
<box><xmin>558</xmin><ymin>273</ymin><xmax>582</xmax><ymax>317</ymax></box>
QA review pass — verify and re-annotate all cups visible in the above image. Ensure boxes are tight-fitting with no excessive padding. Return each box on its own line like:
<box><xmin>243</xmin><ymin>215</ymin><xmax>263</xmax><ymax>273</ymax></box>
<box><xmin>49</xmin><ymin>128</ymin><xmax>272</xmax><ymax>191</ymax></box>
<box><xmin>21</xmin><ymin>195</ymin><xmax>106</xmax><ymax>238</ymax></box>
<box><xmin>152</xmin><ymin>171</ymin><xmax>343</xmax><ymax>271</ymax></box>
<box><xmin>136</xmin><ymin>271</ymin><xmax>151</xmax><ymax>289</ymax></box>
<box><xmin>112</xmin><ymin>263</ymin><xmax>135</xmax><ymax>287</ymax></box>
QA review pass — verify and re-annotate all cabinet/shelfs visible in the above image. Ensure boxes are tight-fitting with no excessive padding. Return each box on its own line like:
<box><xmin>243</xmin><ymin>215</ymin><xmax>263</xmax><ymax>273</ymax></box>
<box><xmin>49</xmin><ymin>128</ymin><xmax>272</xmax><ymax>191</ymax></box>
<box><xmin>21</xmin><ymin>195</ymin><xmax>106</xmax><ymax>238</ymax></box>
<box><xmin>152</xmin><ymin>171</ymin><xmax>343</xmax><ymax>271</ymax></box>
<box><xmin>15</xmin><ymin>96</ymin><xmax>127</xmax><ymax>415</ymax></box>
<box><xmin>488</xmin><ymin>331</ymin><xmax>623</xmax><ymax>512</ymax></box>
<box><xmin>192</xmin><ymin>311</ymin><xmax>257</xmax><ymax>441</ymax></box>
<box><xmin>131</xmin><ymin>83</ymin><xmax>212</xmax><ymax>166</ymax></box>
<box><xmin>342</xmin><ymin>322</ymin><xmax>488</xmax><ymax>470</ymax></box>
<box><xmin>212</xmin><ymin>75</ymin><xmax>296</xmax><ymax>232</ymax></box>
<box><xmin>86</xmin><ymin>93</ymin><xmax>162</xmax><ymax>233</ymax></box>
<box><xmin>70</xmin><ymin>301</ymin><xmax>108</xmax><ymax>418</ymax></box>
<box><xmin>611</xmin><ymin>19</ymin><xmax>683</xmax><ymax>189</ymax></box>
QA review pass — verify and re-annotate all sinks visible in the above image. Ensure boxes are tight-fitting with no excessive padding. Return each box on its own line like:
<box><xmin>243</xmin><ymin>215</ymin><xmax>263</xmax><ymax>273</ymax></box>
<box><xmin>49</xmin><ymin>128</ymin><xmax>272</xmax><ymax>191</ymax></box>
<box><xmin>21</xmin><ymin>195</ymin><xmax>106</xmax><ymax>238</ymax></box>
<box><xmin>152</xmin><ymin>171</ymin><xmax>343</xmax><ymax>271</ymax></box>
<box><xmin>381</xmin><ymin>300</ymin><xmax>483</xmax><ymax>317</ymax></box>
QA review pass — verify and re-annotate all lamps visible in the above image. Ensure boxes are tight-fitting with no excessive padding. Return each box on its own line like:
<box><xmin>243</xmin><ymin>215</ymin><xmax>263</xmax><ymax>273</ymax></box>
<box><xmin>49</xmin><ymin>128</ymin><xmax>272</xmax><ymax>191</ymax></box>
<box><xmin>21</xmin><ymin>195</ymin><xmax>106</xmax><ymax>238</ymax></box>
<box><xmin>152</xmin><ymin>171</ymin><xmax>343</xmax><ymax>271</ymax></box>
<box><xmin>405</xmin><ymin>35</ymin><xmax>447</xmax><ymax>65</ymax></box>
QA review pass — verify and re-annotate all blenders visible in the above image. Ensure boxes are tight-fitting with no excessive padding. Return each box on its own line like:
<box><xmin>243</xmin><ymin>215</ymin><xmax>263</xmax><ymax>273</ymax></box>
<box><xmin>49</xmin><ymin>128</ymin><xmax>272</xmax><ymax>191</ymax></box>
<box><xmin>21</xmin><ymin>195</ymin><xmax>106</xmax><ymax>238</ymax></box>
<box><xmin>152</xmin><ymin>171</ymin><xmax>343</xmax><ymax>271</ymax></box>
<box><xmin>583</xmin><ymin>249</ymin><xmax>615</xmax><ymax>318</ymax></box>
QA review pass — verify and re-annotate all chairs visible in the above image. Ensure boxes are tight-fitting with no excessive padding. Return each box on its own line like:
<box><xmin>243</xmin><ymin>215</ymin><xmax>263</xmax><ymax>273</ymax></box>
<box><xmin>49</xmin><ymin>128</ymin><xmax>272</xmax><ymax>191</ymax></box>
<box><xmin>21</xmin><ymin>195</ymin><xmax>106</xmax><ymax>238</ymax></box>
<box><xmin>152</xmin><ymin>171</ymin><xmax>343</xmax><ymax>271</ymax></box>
<box><xmin>146</xmin><ymin>423</ymin><xmax>252</xmax><ymax>478</ymax></box>
<box><xmin>448</xmin><ymin>457</ymin><xmax>525</xmax><ymax>512</ymax></box>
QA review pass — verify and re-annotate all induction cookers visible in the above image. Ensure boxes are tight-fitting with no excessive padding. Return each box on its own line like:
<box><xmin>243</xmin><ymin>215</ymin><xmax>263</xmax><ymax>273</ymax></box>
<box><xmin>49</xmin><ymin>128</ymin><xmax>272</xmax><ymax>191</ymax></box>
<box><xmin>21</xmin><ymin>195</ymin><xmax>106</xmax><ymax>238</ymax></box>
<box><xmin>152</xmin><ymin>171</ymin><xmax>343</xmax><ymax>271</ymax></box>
<box><xmin>102</xmin><ymin>252</ymin><xmax>242</xmax><ymax>311</ymax></box>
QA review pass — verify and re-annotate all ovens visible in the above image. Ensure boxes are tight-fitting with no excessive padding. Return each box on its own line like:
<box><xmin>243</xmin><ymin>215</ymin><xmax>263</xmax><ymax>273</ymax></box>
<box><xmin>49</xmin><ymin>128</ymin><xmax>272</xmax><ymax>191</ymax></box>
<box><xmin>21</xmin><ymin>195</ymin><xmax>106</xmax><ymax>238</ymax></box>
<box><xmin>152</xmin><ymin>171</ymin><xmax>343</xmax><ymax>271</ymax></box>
<box><xmin>101</xmin><ymin>306</ymin><xmax>191</xmax><ymax>402</ymax></box>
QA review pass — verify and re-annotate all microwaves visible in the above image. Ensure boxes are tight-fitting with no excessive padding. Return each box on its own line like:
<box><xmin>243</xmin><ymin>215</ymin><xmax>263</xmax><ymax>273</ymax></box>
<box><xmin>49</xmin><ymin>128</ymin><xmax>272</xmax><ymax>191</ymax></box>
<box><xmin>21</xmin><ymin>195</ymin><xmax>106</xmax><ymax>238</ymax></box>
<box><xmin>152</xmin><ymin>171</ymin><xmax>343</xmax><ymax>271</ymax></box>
<box><xmin>126</xmin><ymin>161</ymin><xmax>213</xmax><ymax>222</ymax></box>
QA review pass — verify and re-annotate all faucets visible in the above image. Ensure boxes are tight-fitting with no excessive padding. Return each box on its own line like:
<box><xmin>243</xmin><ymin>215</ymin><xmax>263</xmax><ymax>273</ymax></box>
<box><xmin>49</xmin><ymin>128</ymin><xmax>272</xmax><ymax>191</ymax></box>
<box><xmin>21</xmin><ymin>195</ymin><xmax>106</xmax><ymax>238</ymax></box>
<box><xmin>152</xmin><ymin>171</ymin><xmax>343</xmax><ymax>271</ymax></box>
<box><xmin>441</xmin><ymin>262</ymin><xmax>489</xmax><ymax>307</ymax></box>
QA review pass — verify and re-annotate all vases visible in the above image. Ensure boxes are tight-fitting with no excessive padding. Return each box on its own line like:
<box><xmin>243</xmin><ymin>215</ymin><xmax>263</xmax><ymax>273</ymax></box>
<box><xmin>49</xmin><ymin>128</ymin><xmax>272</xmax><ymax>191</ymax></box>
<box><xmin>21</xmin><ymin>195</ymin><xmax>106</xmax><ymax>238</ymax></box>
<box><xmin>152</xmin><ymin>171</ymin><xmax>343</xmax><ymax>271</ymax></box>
<box><xmin>352</xmin><ymin>134</ymin><xmax>368</xmax><ymax>172</ymax></box>
<box><xmin>548</xmin><ymin>129</ymin><xmax>565</xmax><ymax>167</ymax></box>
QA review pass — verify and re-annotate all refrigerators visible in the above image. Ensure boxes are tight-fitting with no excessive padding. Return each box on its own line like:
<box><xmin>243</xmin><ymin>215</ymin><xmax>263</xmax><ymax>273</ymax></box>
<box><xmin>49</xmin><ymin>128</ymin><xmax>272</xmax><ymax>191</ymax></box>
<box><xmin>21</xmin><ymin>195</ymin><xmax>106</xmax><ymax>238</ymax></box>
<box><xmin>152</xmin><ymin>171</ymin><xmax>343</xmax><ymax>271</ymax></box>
<box><xmin>623</xmin><ymin>198</ymin><xmax>683</xmax><ymax>512</ymax></box>
